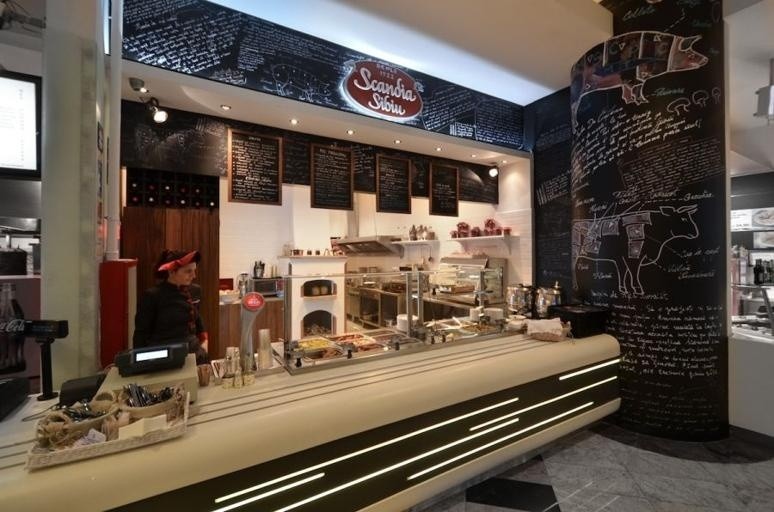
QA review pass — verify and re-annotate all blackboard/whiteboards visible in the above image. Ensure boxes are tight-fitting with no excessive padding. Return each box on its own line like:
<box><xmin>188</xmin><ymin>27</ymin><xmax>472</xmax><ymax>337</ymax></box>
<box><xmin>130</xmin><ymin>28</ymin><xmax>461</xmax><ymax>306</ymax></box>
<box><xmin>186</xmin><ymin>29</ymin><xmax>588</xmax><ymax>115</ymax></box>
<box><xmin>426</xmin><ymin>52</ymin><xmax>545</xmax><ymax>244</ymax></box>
<box><xmin>228</xmin><ymin>128</ymin><xmax>283</xmax><ymax>205</ymax></box>
<box><xmin>376</xmin><ymin>153</ymin><xmax>412</xmax><ymax>214</ymax></box>
<box><xmin>310</xmin><ymin>142</ymin><xmax>354</xmax><ymax>210</ymax></box>
<box><xmin>428</xmin><ymin>161</ymin><xmax>459</xmax><ymax>217</ymax></box>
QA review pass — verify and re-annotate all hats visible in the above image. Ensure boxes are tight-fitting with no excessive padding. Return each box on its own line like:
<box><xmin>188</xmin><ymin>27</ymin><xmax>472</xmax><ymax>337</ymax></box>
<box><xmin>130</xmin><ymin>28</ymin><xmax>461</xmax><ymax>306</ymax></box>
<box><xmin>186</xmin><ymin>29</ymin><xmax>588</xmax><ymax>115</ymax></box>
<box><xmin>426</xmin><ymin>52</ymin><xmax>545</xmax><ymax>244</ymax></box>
<box><xmin>158</xmin><ymin>250</ymin><xmax>202</xmax><ymax>272</ymax></box>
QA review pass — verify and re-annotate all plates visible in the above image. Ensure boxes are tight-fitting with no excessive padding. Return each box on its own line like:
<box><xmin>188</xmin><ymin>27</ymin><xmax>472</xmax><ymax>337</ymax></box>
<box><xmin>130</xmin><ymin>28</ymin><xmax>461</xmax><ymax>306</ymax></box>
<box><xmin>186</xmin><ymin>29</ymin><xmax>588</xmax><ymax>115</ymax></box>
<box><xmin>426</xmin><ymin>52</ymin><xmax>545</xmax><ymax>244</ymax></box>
<box><xmin>360</xmin><ymin>267</ymin><xmax>378</xmax><ymax>273</ymax></box>
<box><xmin>396</xmin><ymin>313</ymin><xmax>418</xmax><ymax>331</ymax></box>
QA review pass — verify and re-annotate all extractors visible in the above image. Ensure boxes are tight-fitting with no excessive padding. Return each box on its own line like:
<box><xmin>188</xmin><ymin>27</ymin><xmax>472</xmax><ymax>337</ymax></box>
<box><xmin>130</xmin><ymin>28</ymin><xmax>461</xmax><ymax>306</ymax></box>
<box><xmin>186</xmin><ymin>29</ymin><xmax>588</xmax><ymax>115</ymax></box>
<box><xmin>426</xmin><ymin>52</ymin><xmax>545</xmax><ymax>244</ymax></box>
<box><xmin>336</xmin><ymin>192</ymin><xmax>404</xmax><ymax>259</ymax></box>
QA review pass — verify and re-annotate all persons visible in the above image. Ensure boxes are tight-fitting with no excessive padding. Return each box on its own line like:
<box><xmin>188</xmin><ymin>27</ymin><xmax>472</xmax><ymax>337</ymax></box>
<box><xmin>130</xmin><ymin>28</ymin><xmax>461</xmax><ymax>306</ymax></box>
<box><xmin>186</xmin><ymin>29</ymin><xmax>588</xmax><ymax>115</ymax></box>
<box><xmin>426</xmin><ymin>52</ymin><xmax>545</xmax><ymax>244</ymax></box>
<box><xmin>134</xmin><ymin>249</ymin><xmax>209</xmax><ymax>354</ymax></box>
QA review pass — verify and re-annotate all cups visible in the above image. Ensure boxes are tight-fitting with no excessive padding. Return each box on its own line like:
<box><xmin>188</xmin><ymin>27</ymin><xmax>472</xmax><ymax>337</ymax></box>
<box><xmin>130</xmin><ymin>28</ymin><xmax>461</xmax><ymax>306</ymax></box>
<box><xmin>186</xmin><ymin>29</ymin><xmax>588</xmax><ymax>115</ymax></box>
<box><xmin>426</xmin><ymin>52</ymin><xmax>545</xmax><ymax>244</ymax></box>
<box><xmin>509</xmin><ymin>314</ymin><xmax>526</xmax><ymax>320</ymax></box>
<box><xmin>306</xmin><ymin>248</ymin><xmax>329</xmax><ymax>256</ymax></box>
<box><xmin>470</xmin><ymin>309</ymin><xmax>504</xmax><ymax>325</ymax></box>
<box><xmin>258</xmin><ymin>330</ymin><xmax>273</xmax><ymax>371</ymax></box>
<box><xmin>196</xmin><ymin>364</ymin><xmax>212</xmax><ymax>387</ymax></box>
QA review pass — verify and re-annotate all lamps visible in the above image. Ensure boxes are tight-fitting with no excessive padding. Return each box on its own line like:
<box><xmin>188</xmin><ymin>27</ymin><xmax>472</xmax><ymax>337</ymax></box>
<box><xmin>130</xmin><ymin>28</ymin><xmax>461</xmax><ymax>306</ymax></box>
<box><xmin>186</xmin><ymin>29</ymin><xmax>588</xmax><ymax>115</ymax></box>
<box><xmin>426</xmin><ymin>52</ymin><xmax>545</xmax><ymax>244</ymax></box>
<box><xmin>488</xmin><ymin>165</ymin><xmax>499</xmax><ymax>177</ymax></box>
<box><xmin>139</xmin><ymin>97</ymin><xmax>168</xmax><ymax>124</ymax></box>
<box><xmin>129</xmin><ymin>77</ymin><xmax>147</xmax><ymax>94</ymax></box>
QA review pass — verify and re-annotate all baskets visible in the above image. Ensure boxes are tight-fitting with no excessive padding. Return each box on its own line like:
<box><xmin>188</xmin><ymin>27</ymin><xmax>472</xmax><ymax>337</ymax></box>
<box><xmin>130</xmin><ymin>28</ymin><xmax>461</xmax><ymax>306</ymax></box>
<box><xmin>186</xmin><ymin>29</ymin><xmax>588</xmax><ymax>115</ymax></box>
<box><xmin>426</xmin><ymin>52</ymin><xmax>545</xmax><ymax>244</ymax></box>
<box><xmin>522</xmin><ymin>321</ymin><xmax>572</xmax><ymax>342</ymax></box>
<box><xmin>43</xmin><ymin>389</ymin><xmax>119</xmax><ymax>444</ymax></box>
<box><xmin>117</xmin><ymin>382</ymin><xmax>185</xmax><ymax>422</ymax></box>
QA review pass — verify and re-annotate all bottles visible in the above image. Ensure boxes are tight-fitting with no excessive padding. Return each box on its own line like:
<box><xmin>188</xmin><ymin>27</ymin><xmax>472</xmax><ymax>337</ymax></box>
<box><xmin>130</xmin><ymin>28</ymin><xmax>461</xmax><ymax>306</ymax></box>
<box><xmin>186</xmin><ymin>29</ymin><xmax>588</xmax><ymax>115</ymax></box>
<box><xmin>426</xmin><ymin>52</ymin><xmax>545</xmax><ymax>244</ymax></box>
<box><xmin>222</xmin><ymin>350</ymin><xmax>255</xmax><ymax>388</ymax></box>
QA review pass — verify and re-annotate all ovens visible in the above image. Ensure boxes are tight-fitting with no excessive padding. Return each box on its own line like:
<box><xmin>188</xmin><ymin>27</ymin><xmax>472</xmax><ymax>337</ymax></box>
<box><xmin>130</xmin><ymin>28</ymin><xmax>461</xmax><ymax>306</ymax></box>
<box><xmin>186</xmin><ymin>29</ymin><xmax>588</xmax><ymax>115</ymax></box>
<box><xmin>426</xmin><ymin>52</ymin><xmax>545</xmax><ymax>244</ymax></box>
<box><xmin>249</xmin><ymin>278</ymin><xmax>278</xmax><ymax>297</ymax></box>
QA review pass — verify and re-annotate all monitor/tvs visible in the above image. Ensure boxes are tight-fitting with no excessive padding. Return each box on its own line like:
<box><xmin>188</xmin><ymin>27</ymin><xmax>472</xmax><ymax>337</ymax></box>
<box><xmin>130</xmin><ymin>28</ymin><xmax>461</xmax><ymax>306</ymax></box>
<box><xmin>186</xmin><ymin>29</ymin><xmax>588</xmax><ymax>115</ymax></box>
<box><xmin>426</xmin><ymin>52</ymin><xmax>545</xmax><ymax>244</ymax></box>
<box><xmin>114</xmin><ymin>341</ymin><xmax>190</xmax><ymax>378</ymax></box>
<box><xmin>0</xmin><ymin>248</ymin><xmax>27</xmax><ymax>276</ymax></box>
<box><xmin>0</xmin><ymin>69</ymin><xmax>42</xmax><ymax>181</ymax></box>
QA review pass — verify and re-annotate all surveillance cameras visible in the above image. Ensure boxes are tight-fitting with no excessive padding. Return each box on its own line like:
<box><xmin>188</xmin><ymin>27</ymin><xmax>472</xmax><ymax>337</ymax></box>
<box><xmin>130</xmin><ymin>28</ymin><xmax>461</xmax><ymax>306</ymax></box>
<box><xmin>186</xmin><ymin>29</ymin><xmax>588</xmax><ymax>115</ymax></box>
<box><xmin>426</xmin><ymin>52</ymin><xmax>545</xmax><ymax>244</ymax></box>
<box><xmin>129</xmin><ymin>78</ymin><xmax>144</xmax><ymax>91</ymax></box>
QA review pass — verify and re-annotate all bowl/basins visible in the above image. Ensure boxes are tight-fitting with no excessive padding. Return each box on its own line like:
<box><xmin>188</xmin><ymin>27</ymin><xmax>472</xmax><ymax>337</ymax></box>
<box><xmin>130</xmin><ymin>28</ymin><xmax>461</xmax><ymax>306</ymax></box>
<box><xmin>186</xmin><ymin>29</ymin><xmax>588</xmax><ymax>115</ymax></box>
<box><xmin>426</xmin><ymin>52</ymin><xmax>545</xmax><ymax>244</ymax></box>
<box><xmin>364</xmin><ymin>282</ymin><xmax>376</xmax><ymax>287</ymax></box>
<box><xmin>220</xmin><ymin>291</ymin><xmax>240</xmax><ymax>304</ymax></box>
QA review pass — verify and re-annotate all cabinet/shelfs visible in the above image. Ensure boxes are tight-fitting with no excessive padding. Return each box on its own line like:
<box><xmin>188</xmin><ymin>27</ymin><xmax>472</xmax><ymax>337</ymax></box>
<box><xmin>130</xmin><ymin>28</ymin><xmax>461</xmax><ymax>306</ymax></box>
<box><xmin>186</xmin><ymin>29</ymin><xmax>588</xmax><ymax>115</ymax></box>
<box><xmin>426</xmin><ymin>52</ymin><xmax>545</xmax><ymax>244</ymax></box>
<box><xmin>345</xmin><ymin>285</ymin><xmax>471</xmax><ymax>329</ymax></box>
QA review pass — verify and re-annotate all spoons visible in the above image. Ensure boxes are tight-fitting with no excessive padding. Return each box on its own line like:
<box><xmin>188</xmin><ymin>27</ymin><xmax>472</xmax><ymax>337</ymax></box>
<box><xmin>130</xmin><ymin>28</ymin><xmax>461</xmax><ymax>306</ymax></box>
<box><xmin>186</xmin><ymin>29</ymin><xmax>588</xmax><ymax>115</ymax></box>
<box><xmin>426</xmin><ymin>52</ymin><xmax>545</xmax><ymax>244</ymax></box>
<box><xmin>22</xmin><ymin>401</ymin><xmax>68</xmax><ymax>421</ymax></box>
